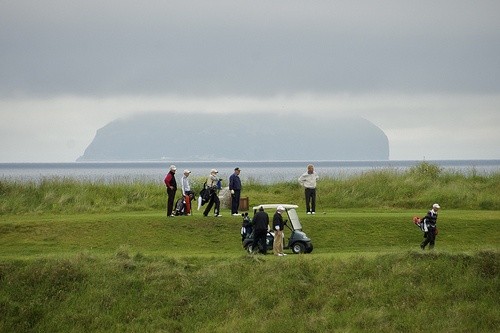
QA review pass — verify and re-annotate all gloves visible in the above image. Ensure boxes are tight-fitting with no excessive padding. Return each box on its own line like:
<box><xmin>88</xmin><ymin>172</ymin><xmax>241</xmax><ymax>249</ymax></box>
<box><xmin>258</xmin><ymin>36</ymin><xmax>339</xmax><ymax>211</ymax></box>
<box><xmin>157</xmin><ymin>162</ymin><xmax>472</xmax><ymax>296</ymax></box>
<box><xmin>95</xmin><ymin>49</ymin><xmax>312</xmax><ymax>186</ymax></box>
<box><xmin>230</xmin><ymin>189</ymin><xmax>235</xmax><ymax>194</ymax></box>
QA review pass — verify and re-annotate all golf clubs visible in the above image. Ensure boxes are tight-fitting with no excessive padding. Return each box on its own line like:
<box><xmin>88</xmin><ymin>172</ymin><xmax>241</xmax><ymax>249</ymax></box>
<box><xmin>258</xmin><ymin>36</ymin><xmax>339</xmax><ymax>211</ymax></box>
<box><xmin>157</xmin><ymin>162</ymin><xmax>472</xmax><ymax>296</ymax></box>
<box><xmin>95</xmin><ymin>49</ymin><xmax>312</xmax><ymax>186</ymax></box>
<box><xmin>316</xmin><ymin>188</ymin><xmax>326</xmax><ymax>214</ymax></box>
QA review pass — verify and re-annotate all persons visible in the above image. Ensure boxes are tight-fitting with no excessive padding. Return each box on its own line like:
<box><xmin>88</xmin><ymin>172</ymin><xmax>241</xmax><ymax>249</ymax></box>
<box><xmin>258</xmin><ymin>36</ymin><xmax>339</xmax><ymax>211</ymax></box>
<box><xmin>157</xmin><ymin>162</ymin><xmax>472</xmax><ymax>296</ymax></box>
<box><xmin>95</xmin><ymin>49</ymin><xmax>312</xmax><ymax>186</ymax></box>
<box><xmin>164</xmin><ymin>165</ymin><xmax>177</xmax><ymax>217</ymax></box>
<box><xmin>298</xmin><ymin>164</ymin><xmax>319</xmax><ymax>214</ymax></box>
<box><xmin>420</xmin><ymin>203</ymin><xmax>441</xmax><ymax>250</ymax></box>
<box><xmin>180</xmin><ymin>169</ymin><xmax>192</xmax><ymax>216</ymax></box>
<box><xmin>250</xmin><ymin>208</ymin><xmax>269</xmax><ymax>255</ymax></box>
<box><xmin>272</xmin><ymin>206</ymin><xmax>287</xmax><ymax>256</ymax></box>
<box><xmin>229</xmin><ymin>167</ymin><xmax>242</xmax><ymax>217</ymax></box>
<box><xmin>203</xmin><ymin>169</ymin><xmax>222</xmax><ymax>217</ymax></box>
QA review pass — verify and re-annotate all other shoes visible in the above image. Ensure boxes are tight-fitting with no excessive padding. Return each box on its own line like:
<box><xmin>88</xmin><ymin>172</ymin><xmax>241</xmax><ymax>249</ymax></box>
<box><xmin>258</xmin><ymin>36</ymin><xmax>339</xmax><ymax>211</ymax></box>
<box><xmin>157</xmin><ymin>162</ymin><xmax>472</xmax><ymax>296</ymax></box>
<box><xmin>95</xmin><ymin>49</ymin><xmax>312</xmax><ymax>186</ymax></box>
<box><xmin>277</xmin><ymin>253</ymin><xmax>283</xmax><ymax>257</ymax></box>
<box><xmin>283</xmin><ymin>253</ymin><xmax>287</xmax><ymax>256</ymax></box>
<box><xmin>312</xmin><ymin>212</ymin><xmax>316</xmax><ymax>214</ymax></box>
<box><xmin>232</xmin><ymin>213</ymin><xmax>241</xmax><ymax>216</ymax></box>
<box><xmin>215</xmin><ymin>215</ymin><xmax>222</xmax><ymax>217</ymax></box>
<box><xmin>307</xmin><ymin>212</ymin><xmax>312</xmax><ymax>214</ymax></box>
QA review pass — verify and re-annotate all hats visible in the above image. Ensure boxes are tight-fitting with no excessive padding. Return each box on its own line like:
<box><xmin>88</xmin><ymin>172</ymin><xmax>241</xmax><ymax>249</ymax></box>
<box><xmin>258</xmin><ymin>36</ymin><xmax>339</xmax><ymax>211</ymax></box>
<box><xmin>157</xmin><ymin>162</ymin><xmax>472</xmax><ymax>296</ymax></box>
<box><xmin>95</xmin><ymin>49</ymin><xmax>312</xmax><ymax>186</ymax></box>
<box><xmin>183</xmin><ymin>169</ymin><xmax>191</xmax><ymax>174</ymax></box>
<box><xmin>277</xmin><ymin>206</ymin><xmax>285</xmax><ymax>210</ymax></box>
<box><xmin>433</xmin><ymin>204</ymin><xmax>440</xmax><ymax>208</ymax></box>
<box><xmin>170</xmin><ymin>165</ymin><xmax>176</xmax><ymax>171</ymax></box>
<box><xmin>210</xmin><ymin>168</ymin><xmax>218</xmax><ymax>173</ymax></box>
<box><xmin>258</xmin><ymin>205</ymin><xmax>265</xmax><ymax>209</ymax></box>
<box><xmin>234</xmin><ymin>167</ymin><xmax>241</xmax><ymax>171</ymax></box>
